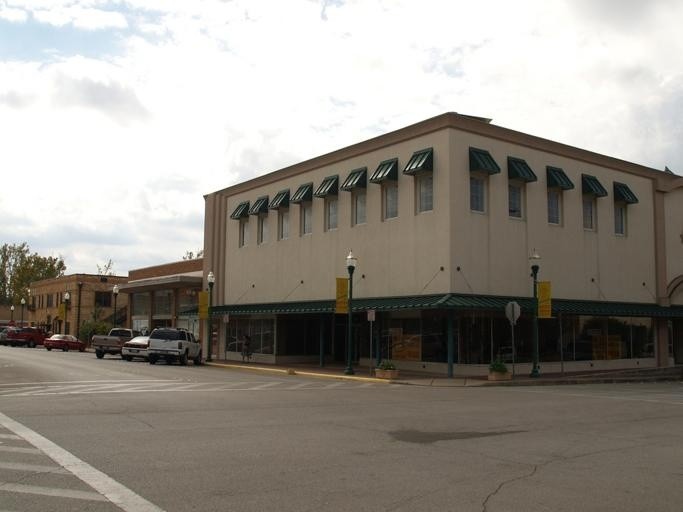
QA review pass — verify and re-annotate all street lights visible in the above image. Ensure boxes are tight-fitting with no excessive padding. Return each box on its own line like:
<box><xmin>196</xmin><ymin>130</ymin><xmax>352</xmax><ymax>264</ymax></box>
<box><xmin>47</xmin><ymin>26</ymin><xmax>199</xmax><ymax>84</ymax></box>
<box><xmin>205</xmin><ymin>271</ymin><xmax>216</xmax><ymax>362</ymax></box>
<box><xmin>528</xmin><ymin>247</ymin><xmax>542</xmax><ymax>378</ymax></box>
<box><xmin>21</xmin><ymin>298</ymin><xmax>25</xmax><ymax>323</ymax></box>
<box><xmin>343</xmin><ymin>248</ymin><xmax>357</xmax><ymax>374</ymax></box>
<box><xmin>113</xmin><ymin>284</ymin><xmax>119</xmax><ymax>328</ymax></box>
<box><xmin>64</xmin><ymin>290</ymin><xmax>70</xmax><ymax>335</ymax></box>
<box><xmin>11</xmin><ymin>305</ymin><xmax>14</xmax><ymax>320</ymax></box>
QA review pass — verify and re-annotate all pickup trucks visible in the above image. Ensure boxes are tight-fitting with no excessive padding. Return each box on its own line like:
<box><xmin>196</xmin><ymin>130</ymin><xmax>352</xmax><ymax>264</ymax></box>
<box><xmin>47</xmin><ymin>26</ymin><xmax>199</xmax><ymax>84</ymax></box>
<box><xmin>91</xmin><ymin>328</ymin><xmax>143</xmax><ymax>358</ymax></box>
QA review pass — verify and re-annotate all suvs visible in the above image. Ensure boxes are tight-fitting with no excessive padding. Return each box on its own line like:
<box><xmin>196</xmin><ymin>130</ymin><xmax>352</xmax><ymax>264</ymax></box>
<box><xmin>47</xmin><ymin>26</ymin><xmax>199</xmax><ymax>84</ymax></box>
<box><xmin>147</xmin><ymin>326</ymin><xmax>203</xmax><ymax>366</ymax></box>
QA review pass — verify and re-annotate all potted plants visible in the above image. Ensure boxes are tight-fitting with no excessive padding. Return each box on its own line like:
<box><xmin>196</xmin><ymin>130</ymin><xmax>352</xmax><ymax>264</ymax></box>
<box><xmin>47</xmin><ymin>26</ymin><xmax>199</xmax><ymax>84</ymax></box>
<box><xmin>376</xmin><ymin>360</ymin><xmax>400</xmax><ymax>379</ymax></box>
<box><xmin>488</xmin><ymin>359</ymin><xmax>511</xmax><ymax>381</ymax></box>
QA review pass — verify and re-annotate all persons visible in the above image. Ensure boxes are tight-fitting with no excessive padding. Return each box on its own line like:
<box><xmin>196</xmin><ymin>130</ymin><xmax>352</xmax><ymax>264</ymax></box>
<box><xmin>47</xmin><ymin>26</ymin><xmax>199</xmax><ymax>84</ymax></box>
<box><xmin>241</xmin><ymin>333</ymin><xmax>251</xmax><ymax>363</ymax></box>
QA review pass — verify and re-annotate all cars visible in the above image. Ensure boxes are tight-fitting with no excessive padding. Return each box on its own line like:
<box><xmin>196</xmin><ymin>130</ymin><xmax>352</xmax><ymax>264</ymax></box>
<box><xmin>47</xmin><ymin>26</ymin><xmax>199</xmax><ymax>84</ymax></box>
<box><xmin>44</xmin><ymin>334</ymin><xmax>86</xmax><ymax>352</ymax></box>
<box><xmin>122</xmin><ymin>336</ymin><xmax>150</xmax><ymax>361</ymax></box>
<box><xmin>0</xmin><ymin>327</ymin><xmax>49</xmax><ymax>348</ymax></box>
